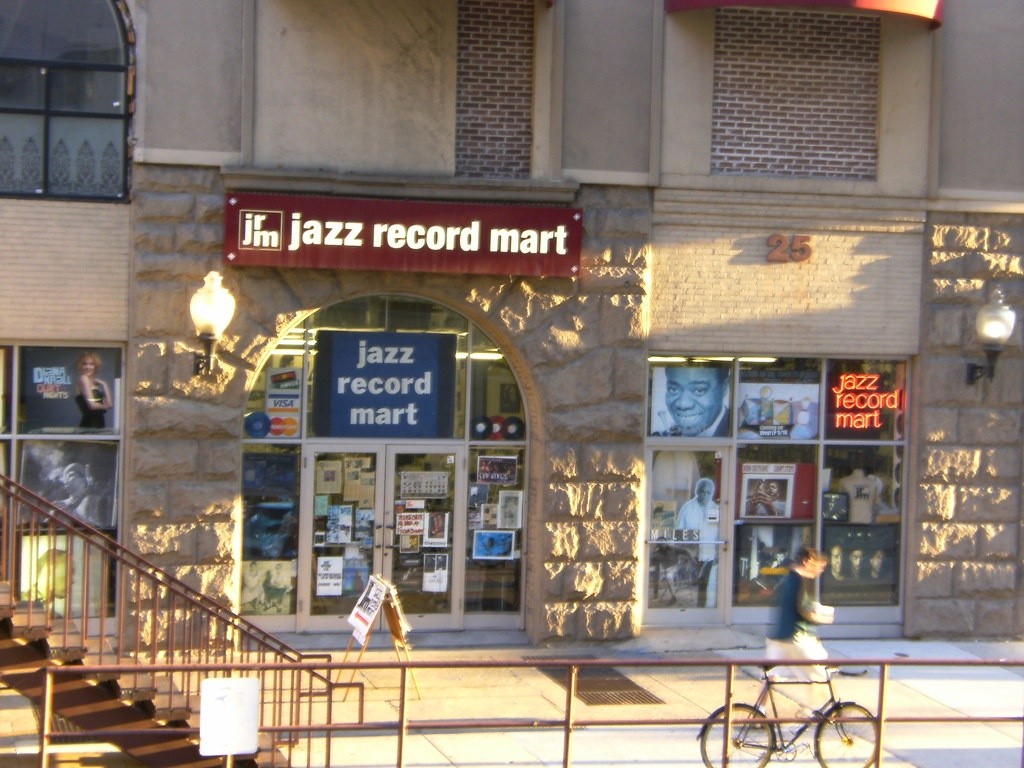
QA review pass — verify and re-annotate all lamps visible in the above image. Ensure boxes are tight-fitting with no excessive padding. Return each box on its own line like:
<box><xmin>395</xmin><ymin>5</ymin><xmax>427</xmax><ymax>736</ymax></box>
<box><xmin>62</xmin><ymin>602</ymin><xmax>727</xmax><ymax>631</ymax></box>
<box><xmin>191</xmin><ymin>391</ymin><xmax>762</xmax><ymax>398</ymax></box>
<box><xmin>967</xmin><ymin>288</ymin><xmax>1017</xmax><ymax>386</ymax></box>
<box><xmin>189</xmin><ymin>271</ymin><xmax>236</xmax><ymax>377</ymax></box>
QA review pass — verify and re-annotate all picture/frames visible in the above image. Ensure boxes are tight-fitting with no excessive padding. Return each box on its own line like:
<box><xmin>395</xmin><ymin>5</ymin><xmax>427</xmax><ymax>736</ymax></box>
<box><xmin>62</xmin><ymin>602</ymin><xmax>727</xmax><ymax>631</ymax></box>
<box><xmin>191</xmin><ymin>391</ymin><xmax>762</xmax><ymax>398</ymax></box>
<box><xmin>498</xmin><ymin>490</ymin><xmax>523</xmax><ymax>530</ymax></box>
<box><xmin>472</xmin><ymin>530</ymin><xmax>515</xmax><ymax>560</ymax></box>
<box><xmin>477</xmin><ymin>455</ymin><xmax>518</xmax><ymax>485</ymax></box>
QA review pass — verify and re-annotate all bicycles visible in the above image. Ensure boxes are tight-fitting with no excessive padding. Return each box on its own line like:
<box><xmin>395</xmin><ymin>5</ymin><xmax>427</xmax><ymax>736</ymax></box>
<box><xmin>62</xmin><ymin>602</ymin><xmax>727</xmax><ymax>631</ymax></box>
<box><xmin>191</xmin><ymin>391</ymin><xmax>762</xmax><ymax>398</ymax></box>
<box><xmin>696</xmin><ymin>665</ymin><xmax>878</xmax><ymax>768</ymax></box>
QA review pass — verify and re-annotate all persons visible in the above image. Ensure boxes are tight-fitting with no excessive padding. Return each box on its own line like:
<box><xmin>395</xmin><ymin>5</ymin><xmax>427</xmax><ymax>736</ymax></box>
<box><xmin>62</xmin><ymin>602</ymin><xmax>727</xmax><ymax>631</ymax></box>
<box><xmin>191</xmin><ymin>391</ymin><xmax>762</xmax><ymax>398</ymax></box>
<box><xmin>241</xmin><ymin>561</ymin><xmax>292</xmax><ymax>615</ymax></box>
<box><xmin>650</xmin><ymin>478</ymin><xmax>793</xmax><ymax>608</ymax></box>
<box><xmin>652</xmin><ymin>365</ymin><xmax>730</xmax><ymax>438</ymax></box>
<box><xmin>838</xmin><ymin>468</ymin><xmax>878</xmax><ymax>523</ymax></box>
<box><xmin>21</xmin><ymin>549</ymin><xmax>74</xmax><ymax>615</ymax></box>
<box><xmin>44</xmin><ymin>462</ymin><xmax>107</xmax><ymax>530</ymax></box>
<box><xmin>822</xmin><ymin>544</ymin><xmax>893</xmax><ymax>591</ymax></box>
<box><xmin>755</xmin><ymin>545</ymin><xmax>834</xmax><ymax>728</ymax></box>
<box><xmin>75</xmin><ymin>351</ymin><xmax>112</xmax><ymax>430</ymax></box>
